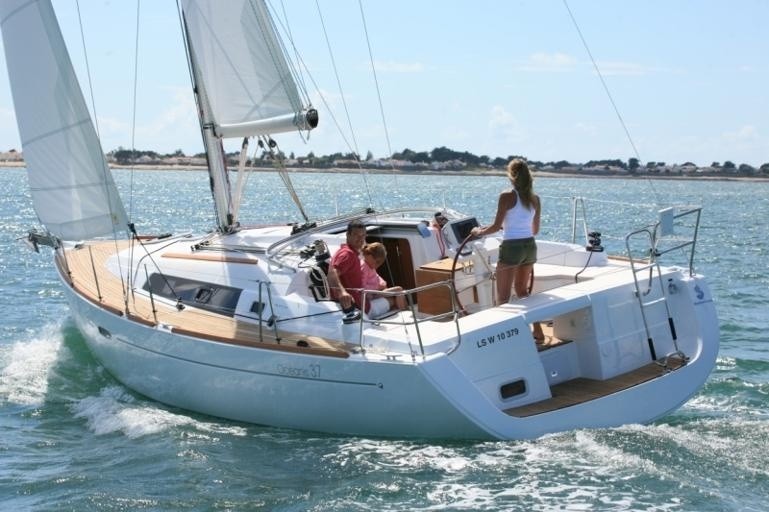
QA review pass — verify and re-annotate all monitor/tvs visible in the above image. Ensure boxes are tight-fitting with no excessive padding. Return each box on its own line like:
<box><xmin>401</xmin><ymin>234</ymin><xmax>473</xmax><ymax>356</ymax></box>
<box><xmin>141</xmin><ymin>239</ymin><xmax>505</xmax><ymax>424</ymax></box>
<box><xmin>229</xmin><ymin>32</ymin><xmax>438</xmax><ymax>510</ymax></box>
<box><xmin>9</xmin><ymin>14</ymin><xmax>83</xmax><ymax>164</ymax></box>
<box><xmin>440</xmin><ymin>215</ymin><xmax>486</xmax><ymax>264</ymax></box>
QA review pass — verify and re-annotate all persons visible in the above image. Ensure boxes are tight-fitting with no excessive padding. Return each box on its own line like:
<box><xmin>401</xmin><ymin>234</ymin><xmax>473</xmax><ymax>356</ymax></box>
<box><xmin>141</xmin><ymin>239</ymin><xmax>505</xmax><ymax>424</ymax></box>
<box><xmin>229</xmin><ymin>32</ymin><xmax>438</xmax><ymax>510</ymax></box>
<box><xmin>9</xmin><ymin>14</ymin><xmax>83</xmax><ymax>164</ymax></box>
<box><xmin>358</xmin><ymin>242</ymin><xmax>408</xmax><ymax>310</ymax></box>
<box><xmin>469</xmin><ymin>158</ymin><xmax>546</xmax><ymax>342</ymax></box>
<box><xmin>326</xmin><ymin>217</ymin><xmax>410</xmax><ymax>319</ymax></box>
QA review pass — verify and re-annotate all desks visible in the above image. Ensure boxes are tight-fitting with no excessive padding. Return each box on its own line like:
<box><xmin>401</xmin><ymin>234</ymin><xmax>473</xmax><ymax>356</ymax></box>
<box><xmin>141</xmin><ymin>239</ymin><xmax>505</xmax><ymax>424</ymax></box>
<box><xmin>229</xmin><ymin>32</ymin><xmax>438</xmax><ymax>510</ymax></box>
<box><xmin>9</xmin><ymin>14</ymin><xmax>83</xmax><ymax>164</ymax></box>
<box><xmin>416</xmin><ymin>258</ymin><xmax>497</xmax><ymax>316</ymax></box>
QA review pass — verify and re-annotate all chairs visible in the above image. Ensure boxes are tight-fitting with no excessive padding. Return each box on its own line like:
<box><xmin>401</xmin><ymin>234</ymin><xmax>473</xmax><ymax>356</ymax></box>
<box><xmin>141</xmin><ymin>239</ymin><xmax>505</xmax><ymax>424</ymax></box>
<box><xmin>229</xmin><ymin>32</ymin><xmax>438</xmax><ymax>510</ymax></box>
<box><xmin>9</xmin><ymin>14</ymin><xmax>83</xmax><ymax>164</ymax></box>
<box><xmin>310</xmin><ymin>262</ymin><xmax>355</xmax><ymax>312</ymax></box>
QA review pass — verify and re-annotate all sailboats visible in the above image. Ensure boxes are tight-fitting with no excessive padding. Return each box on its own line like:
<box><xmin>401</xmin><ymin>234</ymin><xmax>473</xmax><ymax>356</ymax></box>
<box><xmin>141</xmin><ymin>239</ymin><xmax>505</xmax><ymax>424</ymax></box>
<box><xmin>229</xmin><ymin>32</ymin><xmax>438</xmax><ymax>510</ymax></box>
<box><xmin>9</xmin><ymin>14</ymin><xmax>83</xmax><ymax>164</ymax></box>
<box><xmin>0</xmin><ymin>1</ymin><xmax>721</xmax><ymax>441</ymax></box>
